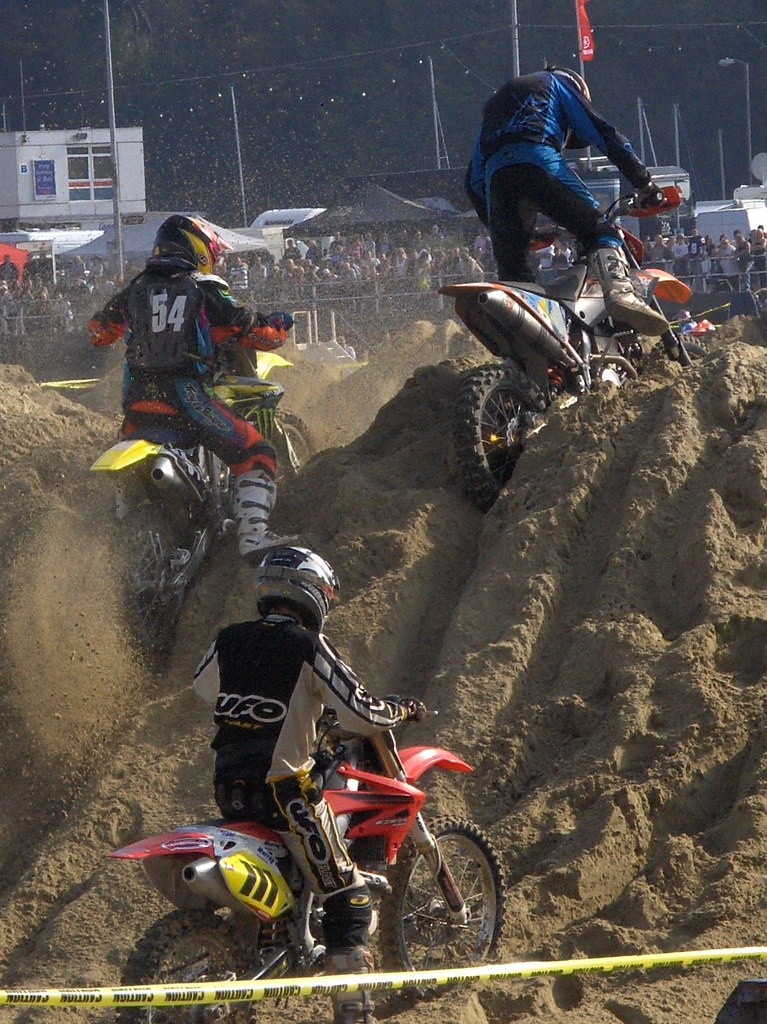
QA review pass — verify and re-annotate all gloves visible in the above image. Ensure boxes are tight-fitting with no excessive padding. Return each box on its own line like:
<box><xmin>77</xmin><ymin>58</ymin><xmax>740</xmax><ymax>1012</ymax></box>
<box><xmin>268</xmin><ymin>311</ymin><xmax>293</xmax><ymax>331</ymax></box>
<box><xmin>640</xmin><ymin>183</ymin><xmax>664</xmax><ymax>210</ymax></box>
<box><xmin>399</xmin><ymin>696</ymin><xmax>427</xmax><ymax>722</ymax></box>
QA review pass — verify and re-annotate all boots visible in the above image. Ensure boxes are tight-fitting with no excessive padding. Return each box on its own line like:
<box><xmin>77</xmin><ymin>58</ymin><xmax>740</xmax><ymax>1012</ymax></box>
<box><xmin>587</xmin><ymin>243</ymin><xmax>669</xmax><ymax>337</ymax></box>
<box><xmin>324</xmin><ymin>946</ymin><xmax>378</xmax><ymax>1024</ymax></box>
<box><xmin>233</xmin><ymin>470</ymin><xmax>292</xmax><ymax>557</ymax></box>
<box><xmin>116</xmin><ymin>483</ymin><xmax>152</xmax><ymax>520</ymax></box>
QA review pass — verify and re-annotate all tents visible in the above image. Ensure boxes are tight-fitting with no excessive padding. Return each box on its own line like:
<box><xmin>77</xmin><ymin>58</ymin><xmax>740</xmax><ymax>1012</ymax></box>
<box><xmin>282</xmin><ymin>178</ymin><xmax>487</xmax><ymax>262</ymax></box>
<box><xmin>0</xmin><ymin>213</ymin><xmax>269</xmax><ymax>285</ymax></box>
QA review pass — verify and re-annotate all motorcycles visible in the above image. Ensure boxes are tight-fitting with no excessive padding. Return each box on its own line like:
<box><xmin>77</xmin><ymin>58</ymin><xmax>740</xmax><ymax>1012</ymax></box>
<box><xmin>108</xmin><ymin>689</ymin><xmax>508</xmax><ymax>1023</ymax></box>
<box><xmin>84</xmin><ymin>313</ymin><xmax>318</xmax><ymax>671</ymax></box>
<box><xmin>438</xmin><ymin>186</ymin><xmax>714</xmax><ymax>514</ymax></box>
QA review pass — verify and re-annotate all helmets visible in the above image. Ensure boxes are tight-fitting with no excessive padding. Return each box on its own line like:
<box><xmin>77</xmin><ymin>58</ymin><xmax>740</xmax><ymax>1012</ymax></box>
<box><xmin>153</xmin><ymin>215</ymin><xmax>222</xmax><ymax>274</ymax></box>
<box><xmin>545</xmin><ymin>64</ymin><xmax>592</xmax><ymax>102</ymax></box>
<box><xmin>255</xmin><ymin>544</ymin><xmax>335</xmax><ymax>635</ymax></box>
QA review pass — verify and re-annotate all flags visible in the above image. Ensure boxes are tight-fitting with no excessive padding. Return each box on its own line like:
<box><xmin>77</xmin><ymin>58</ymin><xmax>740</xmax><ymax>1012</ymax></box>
<box><xmin>576</xmin><ymin>0</ymin><xmax>595</xmax><ymax>62</ymax></box>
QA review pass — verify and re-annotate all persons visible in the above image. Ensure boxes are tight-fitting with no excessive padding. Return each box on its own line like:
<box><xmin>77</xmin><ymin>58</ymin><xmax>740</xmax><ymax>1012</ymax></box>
<box><xmin>0</xmin><ymin>220</ymin><xmax>767</xmax><ymax>361</ymax></box>
<box><xmin>87</xmin><ymin>214</ymin><xmax>303</xmax><ymax>562</ymax></box>
<box><xmin>466</xmin><ymin>62</ymin><xmax>673</xmax><ymax>336</ymax></box>
<box><xmin>192</xmin><ymin>541</ymin><xmax>428</xmax><ymax>1024</ymax></box>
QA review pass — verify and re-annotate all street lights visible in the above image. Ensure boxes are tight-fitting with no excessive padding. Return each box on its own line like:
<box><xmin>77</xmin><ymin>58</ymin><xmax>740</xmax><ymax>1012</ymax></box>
<box><xmin>717</xmin><ymin>58</ymin><xmax>754</xmax><ymax>188</ymax></box>
